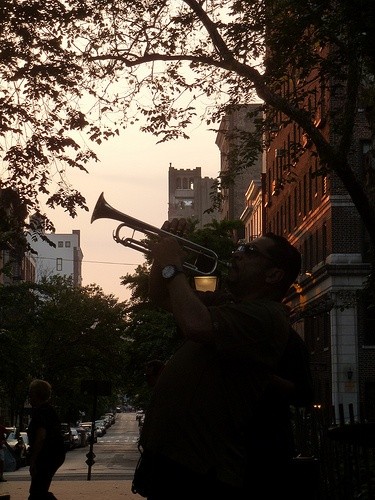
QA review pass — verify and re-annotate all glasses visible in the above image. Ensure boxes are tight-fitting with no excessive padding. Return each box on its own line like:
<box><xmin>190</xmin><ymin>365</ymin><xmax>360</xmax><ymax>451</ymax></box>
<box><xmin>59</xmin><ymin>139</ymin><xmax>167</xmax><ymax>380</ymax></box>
<box><xmin>236</xmin><ymin>244</ymin><xmax>270</xmax><ymax>261</ymax></box>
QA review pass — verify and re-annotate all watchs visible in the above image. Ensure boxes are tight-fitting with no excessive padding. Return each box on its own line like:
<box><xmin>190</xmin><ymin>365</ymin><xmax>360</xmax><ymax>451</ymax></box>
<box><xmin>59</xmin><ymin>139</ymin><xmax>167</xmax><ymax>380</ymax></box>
<box><xmin>159</xmin><ymin>263</ymin><xmax>185</xmax><ymax>282</ymax></box>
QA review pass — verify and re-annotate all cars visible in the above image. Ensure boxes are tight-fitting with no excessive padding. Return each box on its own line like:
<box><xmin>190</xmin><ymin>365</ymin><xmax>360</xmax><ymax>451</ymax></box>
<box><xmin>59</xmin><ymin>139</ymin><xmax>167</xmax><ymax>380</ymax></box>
<box><xmin>0</xmin><ymin>405</ymin><xmax>146</xmax><ymax>470</ymax></box>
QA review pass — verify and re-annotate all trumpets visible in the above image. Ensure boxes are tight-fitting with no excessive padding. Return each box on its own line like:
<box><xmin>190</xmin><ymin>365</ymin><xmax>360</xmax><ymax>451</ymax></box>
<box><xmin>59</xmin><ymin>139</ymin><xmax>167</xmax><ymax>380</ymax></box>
<box><xmin>89</xmin><ymin>191</ymin><xmax>233</xmax><ymax>276</ymax></box>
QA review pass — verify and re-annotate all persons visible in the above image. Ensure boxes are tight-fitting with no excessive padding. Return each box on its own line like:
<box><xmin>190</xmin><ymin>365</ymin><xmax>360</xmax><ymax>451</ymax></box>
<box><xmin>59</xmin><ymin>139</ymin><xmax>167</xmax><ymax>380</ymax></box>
<box><xmin>26</xmin><ymin>379</ymin><xmax>65</xmax><ymax>500</ymax></box>
<box><xmin>130</xmin><ymin>218</ymin><xmax>302</xmax><ymax>500</ymax></box>
<box><xmin>0</xmin><ymin>424</ymin><xmax>7</xmax><ymax>482</ymax></box>
